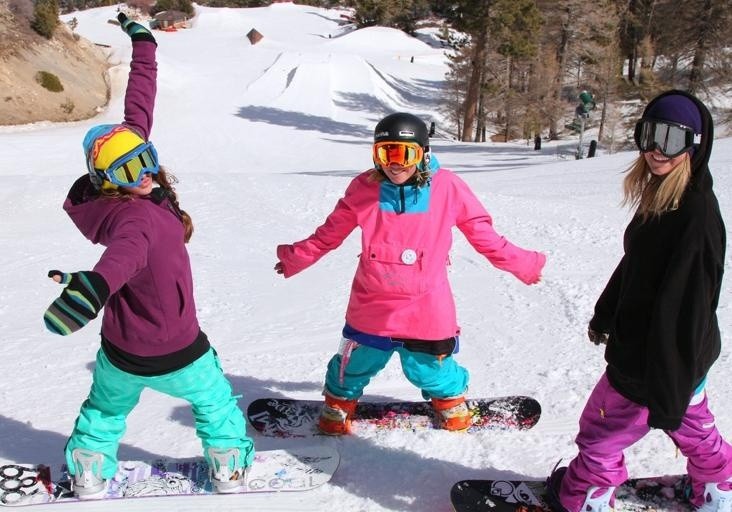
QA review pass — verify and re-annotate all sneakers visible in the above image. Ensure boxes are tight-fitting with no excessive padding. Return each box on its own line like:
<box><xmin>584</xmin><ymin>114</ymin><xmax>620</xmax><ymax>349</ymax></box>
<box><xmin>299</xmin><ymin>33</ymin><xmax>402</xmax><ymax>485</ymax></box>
<box><xmin>682</xmin><ymin>475</ymin><xmax>732</xmax><ymax>512</ymax></box>
<box><xmin>212</xmin><ymin>466</ymin><xmax>243</xmax><ymax>493</ymax></box>
<box><xmin>432</xmin><ymin>396</ymin><xmax>470</xmax><ymax>430</ymax></box>
<box><xmin>549</xmin><ymin>467</ymin><xmax>614</xmax><ymax>512</ymax></box>
<box><xmin>74</xmin><ymin>477</ymin><xmax>107</xmax><ymax>499</ymax></box>
<box><xmin>319</xmin><ymin>396</ymin><xmax>356</xmax><ymax>435</ymax></box>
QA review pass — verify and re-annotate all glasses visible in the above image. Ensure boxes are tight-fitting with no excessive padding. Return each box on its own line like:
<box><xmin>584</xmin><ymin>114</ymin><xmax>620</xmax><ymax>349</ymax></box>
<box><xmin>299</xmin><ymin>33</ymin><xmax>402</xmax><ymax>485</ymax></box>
<box><xmin>91</xmin><ymin>141</ymin><xmax>160</xmax><ymax>188</ymax></box>
<box><xmin>373</xmin><ymin>142</ymin><xmax>424</xmax><ymax>168</ymax></box>
<box><xmin>634</xmin><ymin>119</ymin><xmax>701</xmax><ymax>158</ymax></box>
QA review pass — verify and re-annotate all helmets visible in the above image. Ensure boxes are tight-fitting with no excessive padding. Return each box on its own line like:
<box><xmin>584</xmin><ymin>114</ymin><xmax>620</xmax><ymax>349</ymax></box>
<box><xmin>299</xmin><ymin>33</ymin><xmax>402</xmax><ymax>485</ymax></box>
<box><xmin>642</xmin><ymin>95</ymin><xmax>701</xmax><ymax>150</ymax></box>
<box><xmin>373</xmin><ymin>113</ymin><xmax>429</xmax><ymax>178</ymax></box>
<box><xmin>88</xmin><ymin>126</ymin><xmax>147</xmax><ymax>189</ymax></box>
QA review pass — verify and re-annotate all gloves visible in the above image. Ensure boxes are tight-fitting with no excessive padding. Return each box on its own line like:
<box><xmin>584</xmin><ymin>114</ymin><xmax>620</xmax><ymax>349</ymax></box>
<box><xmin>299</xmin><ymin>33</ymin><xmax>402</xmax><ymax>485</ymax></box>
<box><xmin>647</xmin><ymin>417</ymin><xmax>681</xmax><ymax>432</ymax></box>
<box><xmin>588</xmin><ymin>324</ymin><xmax>605</xmax><ymax>345</ymax></box>
<box><xmin>116</xmin><ymin>13</ymin><xmax>157</xmax><ymax>46</ymax></box>
<box><xmin>44</xmin><ymin>270</ymin><xmax>111</xmax><ymax>336</ymax></box>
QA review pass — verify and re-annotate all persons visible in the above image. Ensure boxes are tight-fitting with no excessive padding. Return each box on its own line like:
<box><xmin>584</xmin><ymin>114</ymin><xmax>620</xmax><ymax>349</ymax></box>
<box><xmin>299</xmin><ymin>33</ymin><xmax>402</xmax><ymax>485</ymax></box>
<box><xmin>548</xmin><ymin>90</ymin><xmax>731</xmax><ymax>512</ymax></box>
<box><xmin>43</xmin><ymin>9</ymin><xmax>255</xmax><ymax>501</ymax></box>
<box><xmin>274</xmin><ymin>113</ymin><xmax>547</xmax><ymax>433</ymax></box>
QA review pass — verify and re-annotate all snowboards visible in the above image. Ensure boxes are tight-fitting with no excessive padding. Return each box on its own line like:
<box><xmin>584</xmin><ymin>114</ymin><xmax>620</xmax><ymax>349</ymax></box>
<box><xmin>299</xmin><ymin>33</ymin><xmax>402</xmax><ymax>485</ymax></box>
<box><xmin>247</xmin><ymin>396</ymin><xmax>541</xmax><ymax>439</ymax></box>
<box><xmin>0</xmin><ymin>443</ymin><xmax>340</xmax><ymax>507</ymax></box>
<box><xmin>450</xmin><ymin>473</ymin><xmax>732</xmax><ymax>511</ymax></box>
